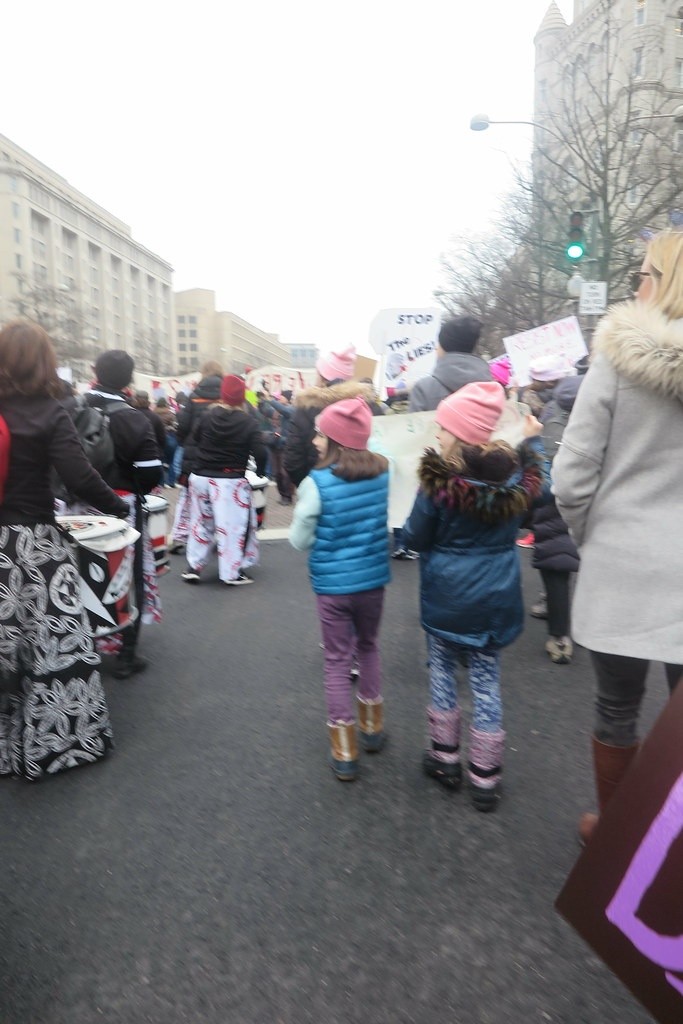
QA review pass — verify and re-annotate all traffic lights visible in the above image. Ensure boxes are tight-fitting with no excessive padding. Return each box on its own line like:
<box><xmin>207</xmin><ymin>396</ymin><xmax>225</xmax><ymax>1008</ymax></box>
<box><xmin>567</xmin><ymin>208</ymin><xmax>584</xmax><ymax>262</ymax></box>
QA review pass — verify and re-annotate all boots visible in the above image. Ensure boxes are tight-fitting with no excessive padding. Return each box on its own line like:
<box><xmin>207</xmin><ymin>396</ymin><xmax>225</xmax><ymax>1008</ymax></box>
<box><xmin>420</xmin><ymin>704</ymin><xmax>463</xmax><ymax>790</ymax></box>
<box><xmin>354</xmin><ymin>693</ymin><xmax>386</xmax><ymax>754</ymax></box>
<box><xmin>466</xmin><ymin>723</ymin><xmax>507</xmax><ymax>813</ymax></box>
<box><xmin>326</xmin><ymin>719</ymin><xmax>360</xmax><ymax>781</ymax></box>
<box><xmin>576</xmin><ymin>737</ymin><xmax>642</xmax><ymax>848</ymax></box>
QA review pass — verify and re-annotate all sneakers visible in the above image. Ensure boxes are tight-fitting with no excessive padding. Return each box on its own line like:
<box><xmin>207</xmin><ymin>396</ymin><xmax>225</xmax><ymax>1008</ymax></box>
<box><xmin>180</xmin><ymin>565</ymin><xmax>201</xmax><ymax>581</ymax></box>
<box><xmin>223</xmin><ymin>570</ymin><xmax>256</xmax><ymax>585</ymax></box>
<box><xmin>515</xmin><ymin>532</ymin><xmax>535</xmax><ymax>550</ymax></box>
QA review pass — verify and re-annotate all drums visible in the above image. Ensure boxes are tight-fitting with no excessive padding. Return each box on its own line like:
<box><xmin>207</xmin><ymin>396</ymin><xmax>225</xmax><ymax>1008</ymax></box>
<box><xmin>142</xmin><ymin>494</ymin><xmax>171</xmax><ymax>577</ymax></box>
<box><xmin>245</xmin><ymin>470</ymin><xmax>269</xmax><ymax>532</ymax></box>
<box><xmin>55</xmin><ymin>513</ymin><xmax>142</xmax><ymax>639</ymax></box>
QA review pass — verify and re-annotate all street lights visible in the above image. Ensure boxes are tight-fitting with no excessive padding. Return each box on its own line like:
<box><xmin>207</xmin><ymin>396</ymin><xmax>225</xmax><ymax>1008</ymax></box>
<box><xmin>471</xmin><ymin>116</ymin><xmax>596</xmax><ymax>327</ymax></box>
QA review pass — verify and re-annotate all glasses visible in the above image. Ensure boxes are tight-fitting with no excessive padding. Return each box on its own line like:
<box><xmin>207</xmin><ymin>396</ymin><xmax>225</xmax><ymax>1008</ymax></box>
<box><xmin>630</xmin><ymin>270</ymin><xmax>663</xmax><ymax>292</ymax></box>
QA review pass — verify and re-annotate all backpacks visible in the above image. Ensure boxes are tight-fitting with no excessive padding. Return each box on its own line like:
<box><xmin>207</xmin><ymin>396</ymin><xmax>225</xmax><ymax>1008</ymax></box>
<box><xmin>46</xmin><ymin>394</ymin><xmax>137</xmax><ymax>506</ymax></box>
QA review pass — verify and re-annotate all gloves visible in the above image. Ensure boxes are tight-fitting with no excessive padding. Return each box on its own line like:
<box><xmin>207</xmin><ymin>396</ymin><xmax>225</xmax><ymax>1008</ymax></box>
<box><xmin>111</xmin><ymin>500</ymin><xmax>130</xmax><ymax>520</ymax></box>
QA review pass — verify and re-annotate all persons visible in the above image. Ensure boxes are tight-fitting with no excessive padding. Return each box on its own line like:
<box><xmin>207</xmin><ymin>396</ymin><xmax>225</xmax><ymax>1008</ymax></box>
<box><xmin>0</xmin><ymin>319</ymin><xmax>166</xmax><ymax>781</ymax></box>
<box><xmin>409</xmin><ymin>316</ymin><xmax>495</xmax><ymax>412</ymax></box>
<box><xmin>550</xmin><ymin>228</ymin><xmax>683</xmax><ymax>845</ymax></box>
<box><xmin>132</xmin><ymin>348</ymin><xmax>420</xmax><ymax>585</ymax></box>
<box><xmin>289</xmin><ymin>398</ymin><xmax>389</xmax><ymax>780</ymax></box>
<box><xmin>401</xmin><ymin>384</ymin><xmax>553</xmax><ymax>810</ymax></box>
<box><xmin>492</xmin><ymin>358</ymin><xmax>589</xmax><ymax>661</ymax></box>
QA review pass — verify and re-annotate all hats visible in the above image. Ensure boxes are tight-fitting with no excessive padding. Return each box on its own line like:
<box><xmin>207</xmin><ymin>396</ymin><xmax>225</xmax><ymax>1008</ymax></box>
<box><xmin>434</xmin><ymin>380</ymin><xmax>506</xmax><ymax>447</ymax></box>
<box><xmin>437</xmin><ymin>314</ymin><xmax>481</xmax><ymax>354</ymax></box>
<box><xmin>314</xmin><ymin>345</ymin><xmax>357</xmax><ymax>382</ymax></box>
<box><xmin>94</xmin><ymin>350</ymin><xmax>135</xmax><ymax>390</ymax></box>
<box><xmin>280</xmin><ymin>390</ymin><xmax>293</xmax><ymax>401</ymax></box>
<box><xmin>529</xmin><ymin>355</ymin><xmax>565</xmax><ymax>381</ymax></box>
<box><xmin>314</xmin><ymin>394</ymin><xmax>373</xmax><ymax>451</ymax></box>
<box><xmin>488</xmin><ymin>356</ymin><xmax>513</xmax><ymax>386</ymax></box>
<box><xmin>220</xmin><ymin>375</ymin><xmax>246</xmax><ymax>405</ymax></box>
<box><xmin>574</xmin><ymin>355</ymin><xmax>590</xmax><ymax>370</ymax></box>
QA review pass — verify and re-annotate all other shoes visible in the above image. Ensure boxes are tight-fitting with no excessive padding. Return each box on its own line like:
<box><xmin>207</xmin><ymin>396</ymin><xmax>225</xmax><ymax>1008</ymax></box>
<box><xmin>268</xmin><ymin>481</ymin><xmax>277</xmax><ymax>487</ymax></box>
<box><xmin>529</xmin><ymin>602</ymin><xmax>549</xmax><ymax>619</ymax></box>
<box><xmin>277</xmin><ymin>500</ymin><xmax>292</xmax><ymax>506</ymax></box>
<box><xmin>543</xmin><ymin>635</ymin><xmax>574</xmax><ymax>665</ymax></box>
<box><xmin>110</xmin><ymin>653</ymin><xmax>149</xmax><ymax>680</ymax></box>
